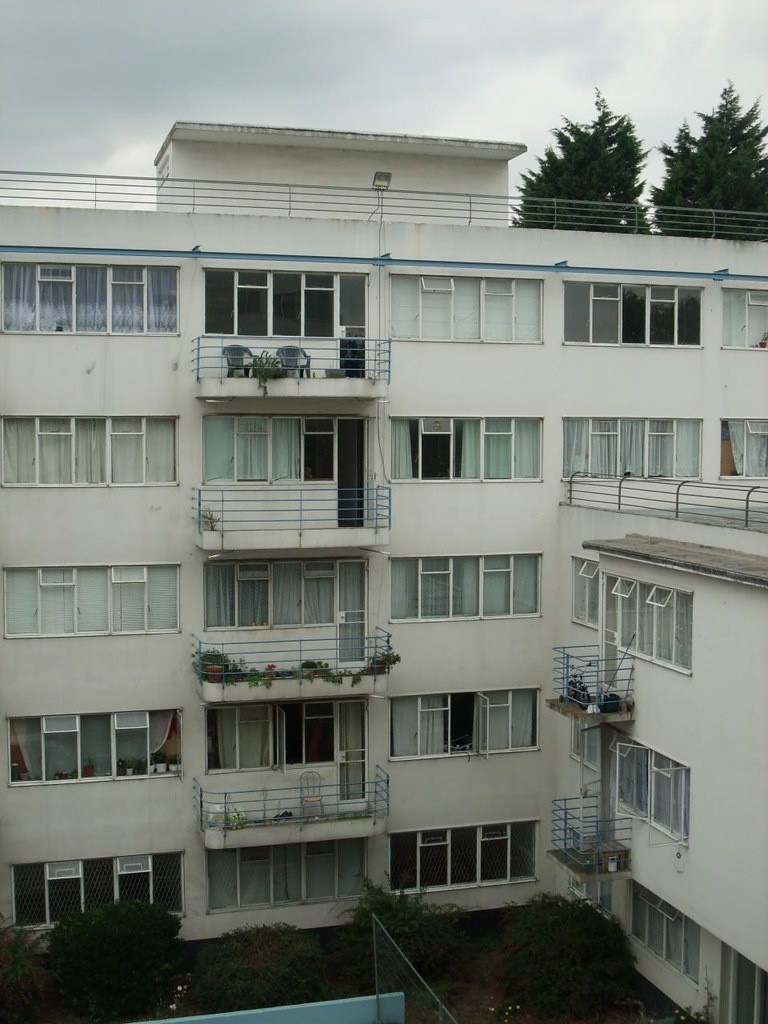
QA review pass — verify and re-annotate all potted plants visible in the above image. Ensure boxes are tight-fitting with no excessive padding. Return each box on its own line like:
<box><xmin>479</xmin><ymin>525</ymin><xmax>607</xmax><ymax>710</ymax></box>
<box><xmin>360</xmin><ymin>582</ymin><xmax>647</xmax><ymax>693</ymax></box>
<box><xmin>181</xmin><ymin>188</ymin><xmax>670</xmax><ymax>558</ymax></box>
<box><xmin>116</xmin><ymin>751</ymin><xmax>180</xmax><ymax>777</ymax></box>
<box><xmin>83</xmin><ymin>755</ymin><xmax>96</xmax><ymax>778</ymax></box>
<box><xmin>299</xmin><ymin>648</ymin><xmax>401</xmax><ymax>686</ymax></box>
<box><xmin>191</xmin><ymin>648</ymin><xmax>260</xmax><ymax>687</ymax></box>
<box><xmin>250</xmin><ymin>350</ymin><xmax>284</xmax><ymax>397</ymax></box>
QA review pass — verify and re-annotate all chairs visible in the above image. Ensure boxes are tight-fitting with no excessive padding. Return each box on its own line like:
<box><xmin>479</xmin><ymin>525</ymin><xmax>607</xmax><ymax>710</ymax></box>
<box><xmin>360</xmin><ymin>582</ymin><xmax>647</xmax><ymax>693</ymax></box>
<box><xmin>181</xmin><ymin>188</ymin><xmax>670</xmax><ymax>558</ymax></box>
<box><xmin>300</xmin><ymin>771</ymin><xmax>324</xmax><ymax>816</ymax></box>
<box><xmin>276</xmin><ymin>346</ymin><xmax>311</xmax><ymax>377</ymax></box>
<box><xmin>223</xmin><ymin>345</ymin><xmax>259</xmax><ymax>379</ymax></box>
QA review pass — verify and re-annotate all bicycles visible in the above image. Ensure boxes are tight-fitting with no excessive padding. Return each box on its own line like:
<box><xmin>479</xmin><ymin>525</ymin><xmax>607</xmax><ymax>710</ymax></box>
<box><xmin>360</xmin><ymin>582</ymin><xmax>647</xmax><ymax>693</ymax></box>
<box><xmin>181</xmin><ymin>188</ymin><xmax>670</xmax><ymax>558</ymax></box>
<box><xmin>566</xmin><ymin>661</ymin><xmax>592</xmax><ymax>710</ymax></box>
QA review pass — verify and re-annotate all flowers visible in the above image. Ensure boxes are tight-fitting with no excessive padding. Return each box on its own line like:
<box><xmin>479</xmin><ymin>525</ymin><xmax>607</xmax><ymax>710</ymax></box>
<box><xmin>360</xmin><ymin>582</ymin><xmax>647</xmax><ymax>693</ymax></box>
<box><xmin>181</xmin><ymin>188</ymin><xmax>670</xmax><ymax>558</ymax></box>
<box><xmin>223</xmin><ymin>808</ymin><xmax>246</xmax><ymax>827</ymax></box>
<box><xmin>267</xmin><ymin>664</ymin><xmax>275</xmax><ymax>670</ymax></box>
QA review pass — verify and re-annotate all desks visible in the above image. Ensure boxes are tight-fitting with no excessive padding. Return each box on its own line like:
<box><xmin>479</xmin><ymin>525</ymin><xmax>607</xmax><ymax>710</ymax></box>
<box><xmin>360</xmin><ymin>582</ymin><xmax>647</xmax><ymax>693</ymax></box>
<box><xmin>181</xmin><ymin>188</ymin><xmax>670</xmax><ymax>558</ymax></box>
<box><xmin>592</xmin><ymin>841</ymin><xmax>630</xmax><ymax>870</ymax></box>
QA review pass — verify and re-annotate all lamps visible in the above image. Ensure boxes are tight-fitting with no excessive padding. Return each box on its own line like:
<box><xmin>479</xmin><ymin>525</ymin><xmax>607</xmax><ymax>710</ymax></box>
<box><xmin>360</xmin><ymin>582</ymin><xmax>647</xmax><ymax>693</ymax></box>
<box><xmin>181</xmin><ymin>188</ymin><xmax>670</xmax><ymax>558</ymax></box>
<box><xmin>372</xmin><ymin>171</ymin><xmax>392</xmax><ymax>188</ymax></box>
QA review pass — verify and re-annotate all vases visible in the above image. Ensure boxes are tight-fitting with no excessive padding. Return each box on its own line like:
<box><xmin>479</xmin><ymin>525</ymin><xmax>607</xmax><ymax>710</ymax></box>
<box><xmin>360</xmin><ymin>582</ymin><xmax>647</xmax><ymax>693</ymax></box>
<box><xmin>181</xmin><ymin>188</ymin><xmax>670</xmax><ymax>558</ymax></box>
<box><xmin>264</xmin><ymin>672</ymin><xmax>275</xmax><ymax>679</ymax></box>
